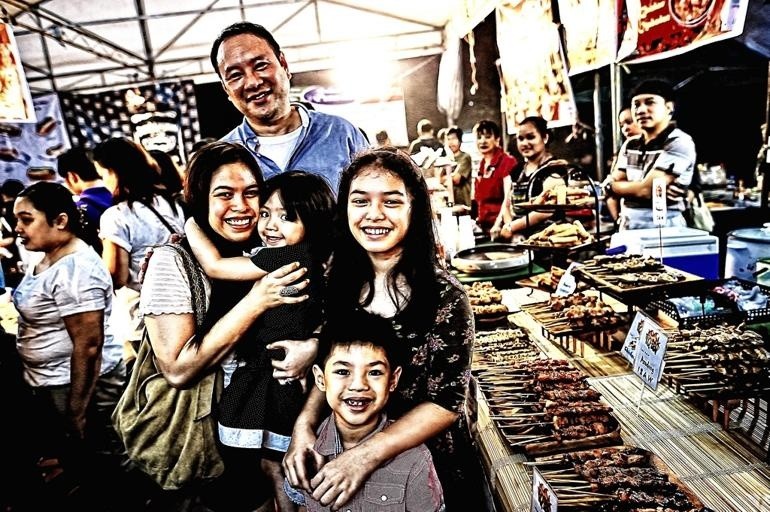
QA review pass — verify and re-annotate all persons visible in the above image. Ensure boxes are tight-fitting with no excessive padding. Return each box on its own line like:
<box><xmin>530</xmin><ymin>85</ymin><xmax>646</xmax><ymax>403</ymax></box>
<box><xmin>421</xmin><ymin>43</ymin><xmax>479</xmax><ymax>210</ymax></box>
<box><xmin>0</xmin><ymin>21</ymin><xmax>703</xmax><ymax>510</ymax></box>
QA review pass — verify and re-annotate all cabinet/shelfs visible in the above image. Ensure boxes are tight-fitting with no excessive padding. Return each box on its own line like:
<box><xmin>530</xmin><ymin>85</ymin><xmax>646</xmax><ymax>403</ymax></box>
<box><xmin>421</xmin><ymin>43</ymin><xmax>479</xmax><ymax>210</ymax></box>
<box><xmin>520</xmin><ymin>257</ymin><xmax>703</xmax><ymax>356</ymax></box>
<box><xmin>526</xmin><ymin>162</ymin><xmax>603</xmax><ymax>299</ymax></box>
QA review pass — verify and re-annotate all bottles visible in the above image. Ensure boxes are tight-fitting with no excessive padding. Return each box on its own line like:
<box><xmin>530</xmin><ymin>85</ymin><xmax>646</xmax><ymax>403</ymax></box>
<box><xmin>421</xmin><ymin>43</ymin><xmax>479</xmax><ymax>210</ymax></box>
<box><xmin>510</xmin><ymin>181</ymin><xmax>530</xmax><ymax>203</ymax></box>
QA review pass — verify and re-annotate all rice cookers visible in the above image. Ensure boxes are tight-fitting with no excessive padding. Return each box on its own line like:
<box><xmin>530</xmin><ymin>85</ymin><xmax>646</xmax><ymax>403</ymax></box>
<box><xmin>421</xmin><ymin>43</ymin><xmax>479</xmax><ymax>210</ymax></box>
<box><xmin>724</xmin><ymin>226</ymin><xmax>770</xmax><ymax>287</ymax></box>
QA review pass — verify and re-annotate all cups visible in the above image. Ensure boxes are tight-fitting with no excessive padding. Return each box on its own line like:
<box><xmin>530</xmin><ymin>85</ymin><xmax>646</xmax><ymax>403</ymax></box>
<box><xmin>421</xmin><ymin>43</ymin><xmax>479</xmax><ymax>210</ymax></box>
<box><xmin>437</xmin><ymin>207</ymin><xmax>477</xmax><ymax>258</ymax></box>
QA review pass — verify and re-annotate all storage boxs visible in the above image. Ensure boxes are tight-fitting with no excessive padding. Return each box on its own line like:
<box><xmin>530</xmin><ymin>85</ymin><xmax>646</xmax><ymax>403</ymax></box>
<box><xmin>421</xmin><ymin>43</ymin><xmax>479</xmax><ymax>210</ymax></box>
<box><xmin>608</xmin><ymin>228</ymin><xmax>720</xmax><ymax>285</ymax></box>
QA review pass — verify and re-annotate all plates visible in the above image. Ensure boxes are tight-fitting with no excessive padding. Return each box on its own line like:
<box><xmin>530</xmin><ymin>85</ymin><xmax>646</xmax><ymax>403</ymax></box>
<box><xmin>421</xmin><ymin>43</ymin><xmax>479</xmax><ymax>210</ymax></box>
<box><xmin>668</xmin><ymin>0</ymin><xmax>717</xmax><ymax>28</ymax></box>
<box><xmin>514</xmin><ymin>202</ymin><xmax>597</xmax><ymax>210</ymax></box>
<box><xmin>41</xmin><ymin>141</ymin><xmax>68</xmax><ymax>159</ymax></box>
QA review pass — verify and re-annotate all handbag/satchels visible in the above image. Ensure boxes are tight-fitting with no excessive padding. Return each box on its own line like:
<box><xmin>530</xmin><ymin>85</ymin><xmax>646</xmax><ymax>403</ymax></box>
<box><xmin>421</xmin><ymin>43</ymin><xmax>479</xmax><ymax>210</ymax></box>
<box><xmin>110</xmin><ymin>327</ymin><xmax>225</xmax><ymax>499</ymax></box>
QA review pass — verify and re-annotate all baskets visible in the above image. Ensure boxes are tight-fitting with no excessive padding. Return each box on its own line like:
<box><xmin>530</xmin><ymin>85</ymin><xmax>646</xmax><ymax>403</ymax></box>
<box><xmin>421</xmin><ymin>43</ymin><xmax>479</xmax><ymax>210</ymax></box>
<box><xmin>645</xmin><ymin>275</ymin><xmax>766</xmax><ymax>331</ymax></box>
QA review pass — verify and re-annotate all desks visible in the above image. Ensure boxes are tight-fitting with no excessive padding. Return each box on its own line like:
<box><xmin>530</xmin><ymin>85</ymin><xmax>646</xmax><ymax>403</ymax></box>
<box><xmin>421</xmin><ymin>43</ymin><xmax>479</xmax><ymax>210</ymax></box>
<box><xmin>448</xmin><ymin>282</ymin><xmax>769</xmax><ymax>511</ymax></box>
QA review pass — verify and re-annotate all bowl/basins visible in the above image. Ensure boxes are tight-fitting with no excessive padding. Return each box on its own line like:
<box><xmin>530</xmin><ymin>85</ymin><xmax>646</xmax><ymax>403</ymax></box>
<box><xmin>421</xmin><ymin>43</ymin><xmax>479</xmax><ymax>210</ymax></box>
<box><xmin>452</xmin><ymin>243</ymin><xmax>536</xmax><ymax>274</ymax></box>
<box><xmin>25</xmin><ymin>165</ymin><xmax>57</xmax><ymax>182</ymax></box>
